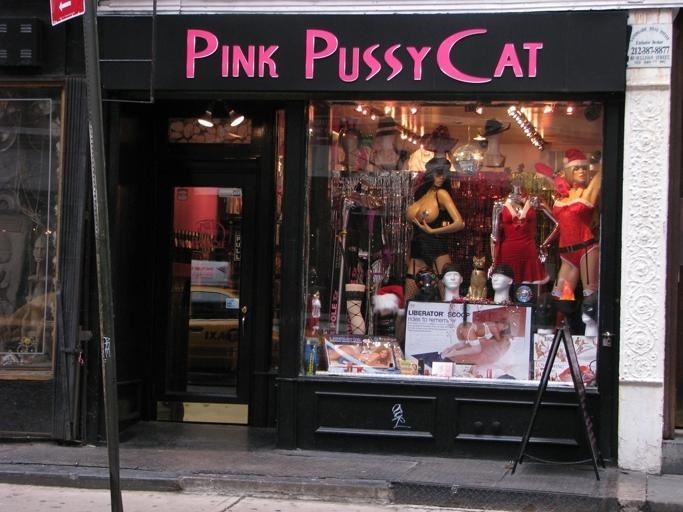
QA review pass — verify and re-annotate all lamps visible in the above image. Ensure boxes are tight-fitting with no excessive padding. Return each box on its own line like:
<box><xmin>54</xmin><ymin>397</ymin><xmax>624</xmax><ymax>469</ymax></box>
<box><xmin>195</xmin><ymin>98</ymin><xmax>245</xmax><ymax>129</ymax></box>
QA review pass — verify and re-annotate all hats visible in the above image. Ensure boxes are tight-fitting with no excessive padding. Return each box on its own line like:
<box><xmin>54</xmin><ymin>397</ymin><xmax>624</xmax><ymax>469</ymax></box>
<box><xmin>424</xmin><ymin>156</ymin><xmax>452</xmax><ymax>172</ymax></box>
<box><xmin>492</xmin><ymin>263</ymin><xmax>515</xmax><ymax>280</ymax></box>
<box><xmin>420</xmin><ymin>125</ymin><xmax>460</xmax><ymax>154</ymax></box>
<box><xmin>374</xmin><ymin>115</ymin><xmax>402</xmax><ymax>137</ymax></box>
<box><xmin>371</xmin><ymin>286</ymin><xmax>407</xmax><ymax>317</ymax></box>
<box><xmin>562</xmin><ymin>149</ymin><xmax>589</xmax><ymax>168</ymax></box>
<box><xmin>478</xmin><ymin>119</ymin><xmax>512</xmax><ymax>138</ymax></box>
<box><xmin>439</xmin><ymin>262</ymin><xmax>464</xmax><ymax>277</ymax></box>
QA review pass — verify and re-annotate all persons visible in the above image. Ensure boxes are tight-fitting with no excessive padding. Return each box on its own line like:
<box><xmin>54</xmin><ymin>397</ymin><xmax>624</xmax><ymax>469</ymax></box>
<box><xmin>335</xmin><ymin>123</ymin><xmax>364</xmax><ymax>172</ymax></box>
<box><xmin>419</xmin><ymin>123</ymin><xmax>463</xmax><ymax>173</ymax></box>
<box><xmin>366</xmin><ymin>114</ymin><xmax>404</xmax><ymax>171</ymax></box>
<box><xmin>572</xmin><ymin>293</ymin><xmax>600</xmax><ymax>339</ymax></box>
<box><xmin>535</xmin><ymin>144</ymin><xmax>599</xmax><ymax>305</ymax></box>
<box><xmin>399</xmin><ymin>157</ymin><xmax>467</xmax><ymax>303</ymax></box>
<box><xmin>324</xmin><ymin>344</ymin><xmax>393</xmax><ymax>368</ymax></box>
<box><xmin>486</xmin><ymin>261</ymin><xmax>517</xmax><ymax>304</ymax></box>
<box><xmin>344</xmin><ymin>282</ymin><xmax>368</xmax><ymax>336</ymax></box>
<box><xmin>432</xmin><ymin>316</ymin><xmax>515</xmax><ymax>359</ymax></box>
<box><xmin>482</xmin><ymin>171</ymin><xmax>561</xmax><ymax>298</ymax></box>
<box><xmin>438</xmin><ymin>261</ymin><xmax>464</xmax><ymax>303</ymax></box>
<box><xmin>478</xmin><ymin>119</ymin><xmax>514</xmax><ymax>168</ymax></box>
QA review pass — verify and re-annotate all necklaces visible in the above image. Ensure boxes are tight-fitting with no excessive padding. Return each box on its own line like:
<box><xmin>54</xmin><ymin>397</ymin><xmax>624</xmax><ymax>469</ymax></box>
<box><xmin>514</xmin><ymin>206</ymin><xmax>522</xmax><ymax>213</ymax></box>
